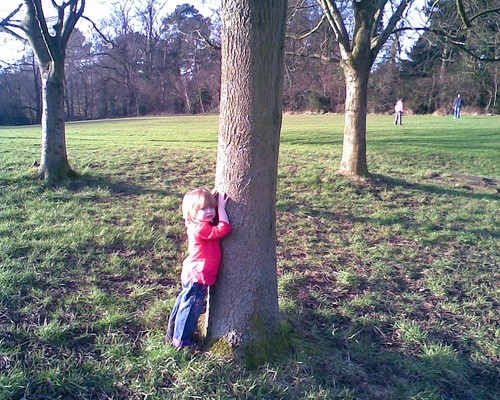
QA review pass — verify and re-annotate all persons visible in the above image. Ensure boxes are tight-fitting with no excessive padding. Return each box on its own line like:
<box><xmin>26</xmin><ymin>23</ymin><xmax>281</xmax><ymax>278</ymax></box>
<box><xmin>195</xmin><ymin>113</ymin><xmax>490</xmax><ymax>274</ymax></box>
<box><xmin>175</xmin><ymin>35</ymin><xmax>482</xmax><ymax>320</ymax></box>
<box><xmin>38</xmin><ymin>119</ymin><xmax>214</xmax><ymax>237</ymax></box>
<box><xmin>394</xmin><ymin>98</ymin><xmax>403</xmax><ymax>126</ymax></box>
<box><xmin>453</xmin><ymin>93</ymin><xmax>465</xmax><ymax>119</ymax></box>
<box><xmin>165</xmin><ymin>188</ymin><xmax>232</xmax><ymax>349</ymax></box>
